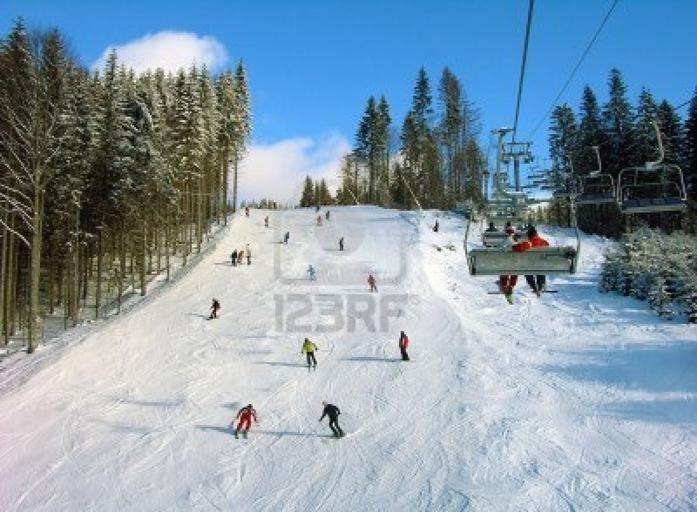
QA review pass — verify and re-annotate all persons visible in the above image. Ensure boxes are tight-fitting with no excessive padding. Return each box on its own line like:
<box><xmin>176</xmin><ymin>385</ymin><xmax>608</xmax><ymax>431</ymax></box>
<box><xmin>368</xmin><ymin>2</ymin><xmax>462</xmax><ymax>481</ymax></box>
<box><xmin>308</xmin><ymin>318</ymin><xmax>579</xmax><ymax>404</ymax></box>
<box><xmin>244</xmin><ymin>243</ymin><xmax>251</xmax><ymax>265</ymax></box>
<box><xmin>318</xmin><ymin>398</ymin><xmax>345</xmax><ymax>439</ymax></box>
<box><xmin>264</xmin><ymin>215</ymin><xmax>269</xmax><ymax>228</ymax></box>
<box><xmin>481</xmin><ymin>219</ymin><xmax>549</xmax><ymax>297</ymax></box>
<box><xmin>316</xmin><ymin>214</ymin><xmax>324</xmax><ymax>227</ymax></box>
<box><xmin>244</xmin><ymin>205</ymin><xmax>249</xmax><ymax>217</ymax></box>
<box><xmin>283</xmin><ymin>230</ymin><xmax>290</xmax><ymax>243</ymax></box>
<box><xmin>338</xmin><ymin>237</ymin><xmax>344</xmax><ymax>252</ymax></box>
<box><xmin>208</xmin><ymin>298</ymin><xmax>220</xmax><ymax>321</ymax></box>
<box><xmin>233</xmin><ymin>402</ymin><xmax>257</xmax><ymax>438</ymax></box>
<box><xmin>367</xmin><ymin>273</ymin><xmax>377</xmax><ymax>293</ymax></box>
<box><xmin>299</xmin><ymin>337</ymin><xmax>319</xmax><ymax>369</ymax></box>
<box><xmin>229</xmin><ymin>248</ymin><xmax>237</xmax><ymax>266</ymax></box>
<box><xmin>305</xmin><ymin>263</ymin><xmax>316</xmax><ymax>281</ymax></box>
<box><xmin>238</xmin><ymin>250</ymin><xmax>244</xmax><ymax>264</ymax></box>
<box><xmin>399</xmin><ymin>329</ymin><xmax>410</xmax><ymax>361</ymax></box>
<box><xmin>325</xmin><ymin>210</ymin><xmax>330</xmax><ymax>220</ymax></box>
